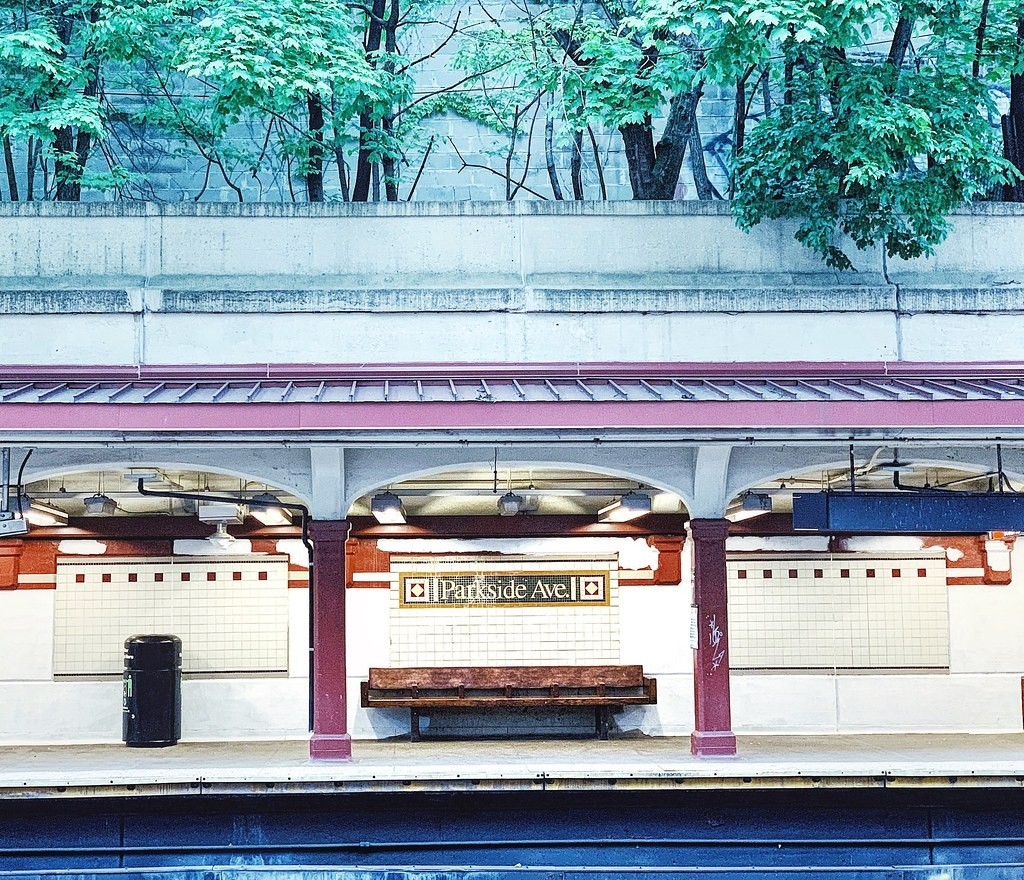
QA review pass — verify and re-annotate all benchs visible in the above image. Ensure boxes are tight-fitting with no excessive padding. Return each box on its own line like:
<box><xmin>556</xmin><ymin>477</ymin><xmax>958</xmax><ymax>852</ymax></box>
<box><xmin>360</xmin><ymin>666</ymin><xmax>658</xmax><ymax>742</ymax></box>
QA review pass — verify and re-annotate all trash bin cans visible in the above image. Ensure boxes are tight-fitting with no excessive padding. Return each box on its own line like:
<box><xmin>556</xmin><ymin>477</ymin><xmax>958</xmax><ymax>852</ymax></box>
<box><xmin>122</xmin><ymin>633</ymin><xmax>183</xmax><ymax>748</ymax></box>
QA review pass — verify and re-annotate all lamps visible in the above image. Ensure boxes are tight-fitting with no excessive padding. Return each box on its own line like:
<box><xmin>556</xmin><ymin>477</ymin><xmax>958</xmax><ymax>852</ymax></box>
<box><xmin>247</xmin><ymin>488</ymin><xmax>292</xmax><ymax>526</ymax></box>
<box><xmin>724</xmin><ymin>491</ymin><xmax>772</xmax><ymax>522</ymax></box>
<box><xmin>597</xmin><ymin>490</ymin><xmax>652</xmax><ymax>523</ymax></box>
<box><xmin>371</xmin><ymin>488</ymin><xmax>407</xmax><ymax>525</ymax></box>
<box><xmin>83</xmin><ymin>493</ymin><xmax>116</xmax><ymax>515</ymax></box>
<box><xmin>0</xmin><ymin>480</ymin><xmax>69</xmax><ymax>527</ymax></box>
<box><xmin>496</xmin><ymin>483</ymin><xmax>521</xmax><ymax>516</ymax></box>
